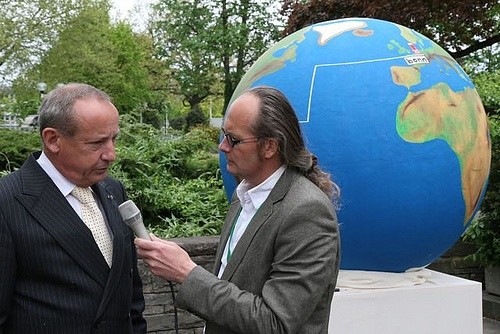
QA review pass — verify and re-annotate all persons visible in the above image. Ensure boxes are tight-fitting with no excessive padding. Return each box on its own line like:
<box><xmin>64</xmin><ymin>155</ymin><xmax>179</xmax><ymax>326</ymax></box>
<box><xmin>134</xmin><ymin>87</ymin><xmax>341</xmax><ymax>334</ymax></box>
<box><xmin>0</xmin><ymin>83</ymin><xmax>147</xmax><ymax>334</ymax></box>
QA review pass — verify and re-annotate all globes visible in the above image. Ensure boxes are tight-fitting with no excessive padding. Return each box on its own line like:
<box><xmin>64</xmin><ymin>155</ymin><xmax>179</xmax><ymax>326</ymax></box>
<box><xmin>217</xmin><ymin>17</ymin><xmax>493</xmax><ymax>334</ymax></box>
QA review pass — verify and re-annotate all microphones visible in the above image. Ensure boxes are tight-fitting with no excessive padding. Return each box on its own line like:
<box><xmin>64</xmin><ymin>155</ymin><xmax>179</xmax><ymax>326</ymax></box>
<box><xmin>117</xmin><ymin>199</ymin><xmax>148</xmax><ymax>244</ymax></box>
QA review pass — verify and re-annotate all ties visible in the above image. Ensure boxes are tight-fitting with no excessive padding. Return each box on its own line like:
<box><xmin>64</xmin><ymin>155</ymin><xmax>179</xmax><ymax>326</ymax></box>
<box><xmin>70</xmin><ymin>186</ymin><xmax>114</xmax><ymax>269</ymax></box>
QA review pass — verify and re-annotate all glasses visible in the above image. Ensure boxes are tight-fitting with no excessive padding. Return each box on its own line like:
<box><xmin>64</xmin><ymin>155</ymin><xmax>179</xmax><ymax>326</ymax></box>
<box><xmin>221</xmin><ymin>127</ymin><xmax>265</xmax><ymax>148</ymax></box>
